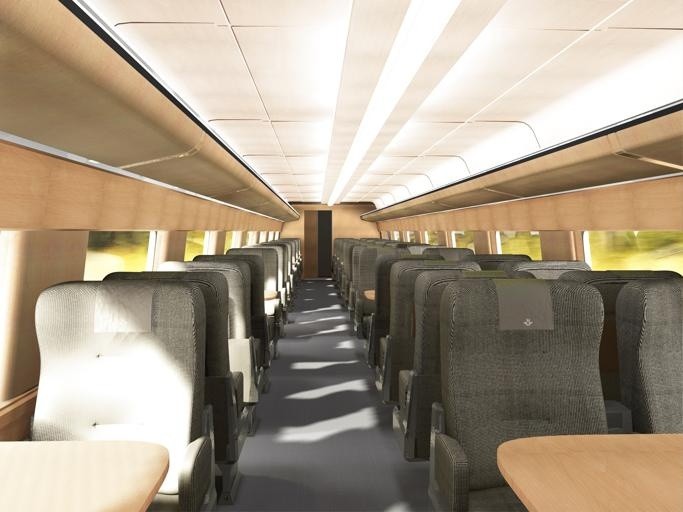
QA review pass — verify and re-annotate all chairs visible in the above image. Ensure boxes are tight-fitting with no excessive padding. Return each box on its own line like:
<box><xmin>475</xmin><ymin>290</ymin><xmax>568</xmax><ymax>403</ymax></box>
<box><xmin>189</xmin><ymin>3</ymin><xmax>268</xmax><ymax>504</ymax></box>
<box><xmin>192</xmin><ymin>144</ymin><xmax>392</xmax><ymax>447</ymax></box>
<box><xmin>28</xmin><ymin>237</ymin><xmax>305</xmax><ymax>512</ymax></box>
<box><xmin>331</xmin><ymin>237</ymin><xmax>682</xmax><ymax>512</ymax></box>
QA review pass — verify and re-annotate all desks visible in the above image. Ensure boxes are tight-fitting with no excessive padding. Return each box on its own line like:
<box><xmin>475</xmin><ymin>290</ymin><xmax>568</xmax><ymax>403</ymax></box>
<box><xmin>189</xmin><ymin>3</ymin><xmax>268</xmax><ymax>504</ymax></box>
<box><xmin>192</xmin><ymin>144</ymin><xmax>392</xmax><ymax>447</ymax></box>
<box><xmin>0</xmin><ymin>439</ymin><xmax>170</xmax><ymax>512</ymax></box>
